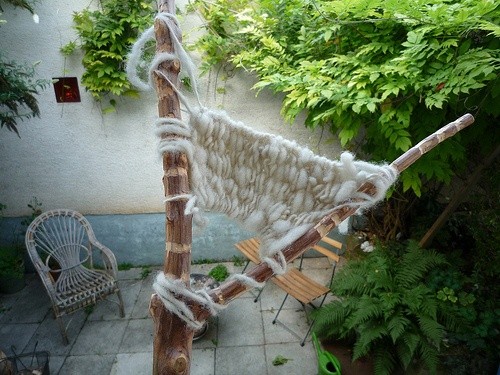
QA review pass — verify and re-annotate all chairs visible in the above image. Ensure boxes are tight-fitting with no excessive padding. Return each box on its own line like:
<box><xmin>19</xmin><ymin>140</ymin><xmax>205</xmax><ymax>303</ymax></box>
<box><xmin>270</xmin><ymin>234</ymin><xmax>343</xmax><ymax>349</ymax></box>
<box><xmin>24</xmin><ymin>208</ymin><xmax>127</xmax><ymax>347</ymax></box>
<box><xmin>233</xmin><ymin>236</ymin><xmax>271</xmax><ymax>303</ymax></box>
